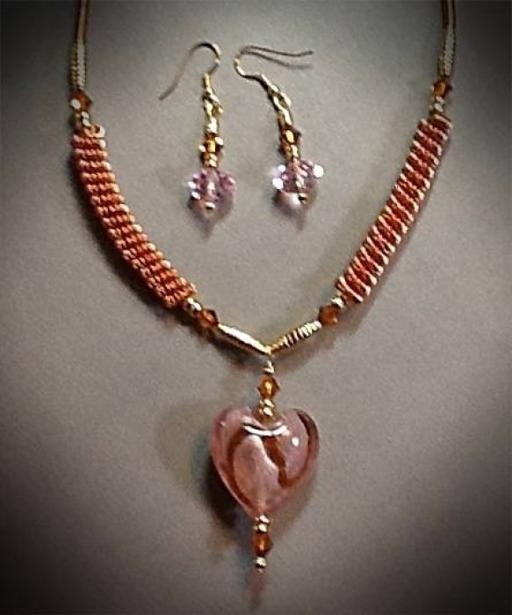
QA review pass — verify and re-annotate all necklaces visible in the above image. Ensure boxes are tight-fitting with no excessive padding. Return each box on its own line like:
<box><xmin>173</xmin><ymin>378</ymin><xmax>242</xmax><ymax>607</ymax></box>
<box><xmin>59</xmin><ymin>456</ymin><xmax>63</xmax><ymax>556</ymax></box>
<box><xmin>68</xmin><ymin>0</ymin><xmax>459</xmax><ymax>590</ymax></box>
